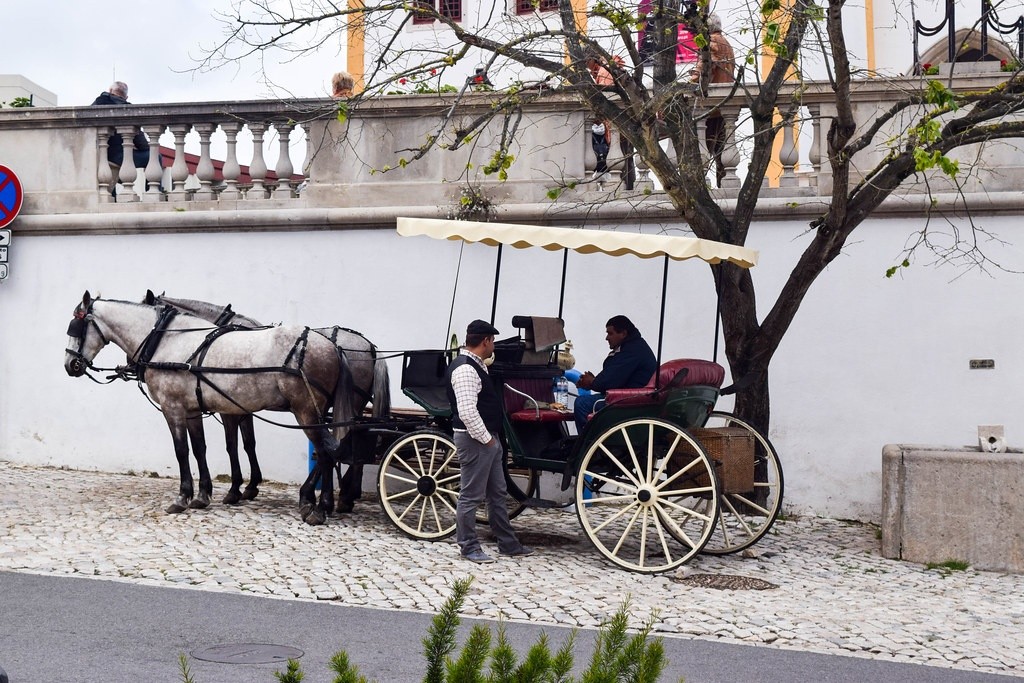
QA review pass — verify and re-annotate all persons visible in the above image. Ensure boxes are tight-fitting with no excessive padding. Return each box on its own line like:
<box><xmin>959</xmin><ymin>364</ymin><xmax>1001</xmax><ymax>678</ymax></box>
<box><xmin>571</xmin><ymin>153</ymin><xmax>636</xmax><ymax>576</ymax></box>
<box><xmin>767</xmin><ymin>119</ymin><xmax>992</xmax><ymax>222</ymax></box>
<box><xmin>92</xmin><ymin>81</ymin><xmax>162</xmax><ymax>200</ymax></box>
<box><xmin>447</xmin><ymin>319</ymin><xmax>534</xmax><ymax>563</ymax></box>
<box><xmin>687</xmin><ymin>13</ymin><xmax>735</xmax><ymax>186</ymax></box>
<box><xmin>581</xmin><ymin>42</ymin><xmax>636</xmax><ymax>188</ymax></box>
<box><xmin>331</xmin><ymin>73</ymin><xmax>354</xmax><ymax>98</ymax></box>
<box><xmin>575</xmin><ymin>316</ymin><xmax>657</xmax><ymax>437</ymax></box>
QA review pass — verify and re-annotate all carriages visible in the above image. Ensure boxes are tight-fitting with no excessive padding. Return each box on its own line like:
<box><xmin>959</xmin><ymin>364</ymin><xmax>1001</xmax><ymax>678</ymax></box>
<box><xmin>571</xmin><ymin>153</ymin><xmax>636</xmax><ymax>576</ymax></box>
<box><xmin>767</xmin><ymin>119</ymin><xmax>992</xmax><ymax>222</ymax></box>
<box><xmin>66</xmin><ymin>216</ymin><xmax>786</xmax><ymax>575</ymax></box>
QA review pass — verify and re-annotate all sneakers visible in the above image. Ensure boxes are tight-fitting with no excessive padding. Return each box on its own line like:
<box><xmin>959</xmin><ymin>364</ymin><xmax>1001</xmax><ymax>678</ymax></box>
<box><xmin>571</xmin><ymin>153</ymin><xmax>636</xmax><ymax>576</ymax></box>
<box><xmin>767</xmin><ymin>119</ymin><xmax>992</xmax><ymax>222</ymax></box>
<box><xmin>499</xmin><ymin>547</ymin><xmax>535</xmax><ymax>556</ymax></box>
<box><xmin>460</xmin><ymin>549</ymin><xmax>494</xmax><ymax>563</ymax></box>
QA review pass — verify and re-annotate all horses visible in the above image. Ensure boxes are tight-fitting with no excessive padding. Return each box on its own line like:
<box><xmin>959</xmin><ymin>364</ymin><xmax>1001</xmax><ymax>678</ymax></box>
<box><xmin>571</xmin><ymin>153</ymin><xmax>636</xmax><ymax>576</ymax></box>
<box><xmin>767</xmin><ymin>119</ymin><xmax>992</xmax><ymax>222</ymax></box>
<box><xmin>63</xmin><ymin>288</ymin><xmax>392</xmax><ymax>527</ymax></box>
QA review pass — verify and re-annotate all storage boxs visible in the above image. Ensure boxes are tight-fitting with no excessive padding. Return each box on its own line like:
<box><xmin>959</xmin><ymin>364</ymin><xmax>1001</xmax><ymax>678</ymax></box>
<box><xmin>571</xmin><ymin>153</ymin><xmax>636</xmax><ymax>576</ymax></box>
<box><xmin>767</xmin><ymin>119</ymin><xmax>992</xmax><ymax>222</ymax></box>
<box><xmin>670</xmin><ymin>427</ymin><xmax>755</xmax><ymax>496</ymax></box>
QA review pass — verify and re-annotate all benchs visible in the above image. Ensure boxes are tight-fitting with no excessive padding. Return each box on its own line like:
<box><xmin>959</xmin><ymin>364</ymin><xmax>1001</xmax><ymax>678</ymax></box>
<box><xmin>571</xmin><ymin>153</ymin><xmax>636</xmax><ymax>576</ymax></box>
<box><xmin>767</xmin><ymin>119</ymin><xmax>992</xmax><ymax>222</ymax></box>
<box><xmin>493</xmin><ymin>315</ymin><xmax>565</xmax><ymax>367</ymax></box>
<box><xmin>588</xmin><ymin>359</ymin><xmax>725</xmax><ymax>422</ymax></box>
<box><xmin>503</xmin><ymin>379</ymin><xmax>576</xmax><ymax>421</ymax></box>
